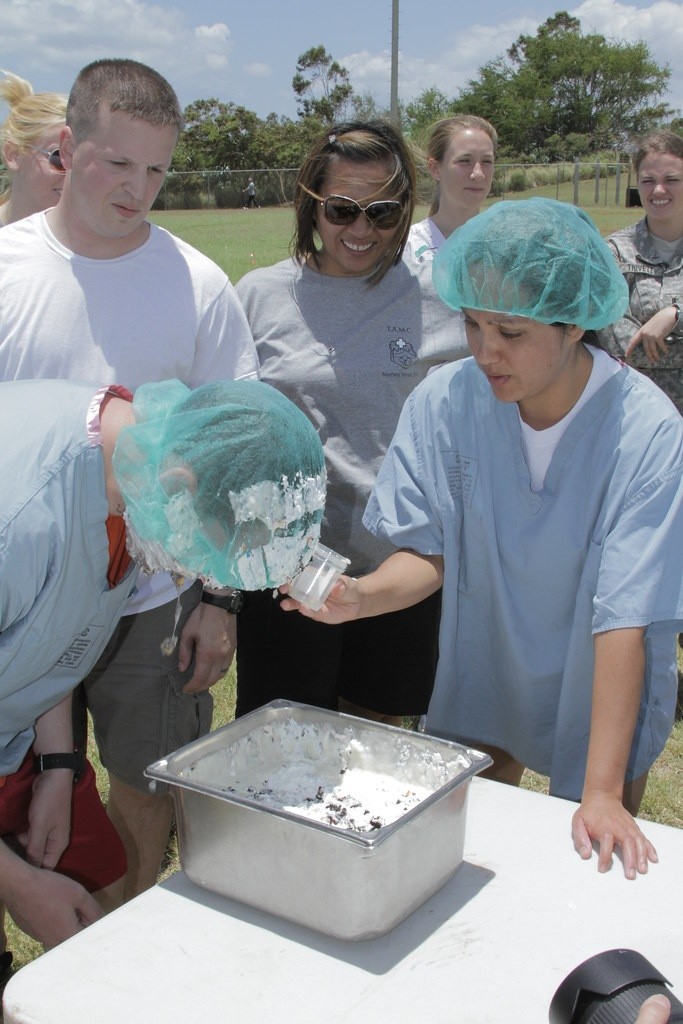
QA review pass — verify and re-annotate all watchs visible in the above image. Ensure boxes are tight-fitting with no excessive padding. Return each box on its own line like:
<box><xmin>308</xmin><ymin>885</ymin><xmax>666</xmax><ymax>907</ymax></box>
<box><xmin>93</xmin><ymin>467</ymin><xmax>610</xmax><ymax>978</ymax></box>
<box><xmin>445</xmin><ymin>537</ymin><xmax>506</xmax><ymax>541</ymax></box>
<box><xmin>200</xmin><ymin>589</ymin><xmax>247</xmax><ymax>614</ymax></box>
<box><xmin>32</xmin><ymin>749</ymin><xmax>88</xmax><ymax>784</ymax></box>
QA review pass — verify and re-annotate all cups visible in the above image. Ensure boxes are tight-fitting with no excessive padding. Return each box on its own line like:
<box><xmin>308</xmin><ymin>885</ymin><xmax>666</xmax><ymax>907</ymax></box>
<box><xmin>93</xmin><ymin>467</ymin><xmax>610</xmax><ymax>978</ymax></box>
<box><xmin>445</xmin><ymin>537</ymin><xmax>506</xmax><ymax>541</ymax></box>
<box><xmin>288</xmin><ymin>542</ymin><xmax>350</xmax><ymax>611</ymax></box>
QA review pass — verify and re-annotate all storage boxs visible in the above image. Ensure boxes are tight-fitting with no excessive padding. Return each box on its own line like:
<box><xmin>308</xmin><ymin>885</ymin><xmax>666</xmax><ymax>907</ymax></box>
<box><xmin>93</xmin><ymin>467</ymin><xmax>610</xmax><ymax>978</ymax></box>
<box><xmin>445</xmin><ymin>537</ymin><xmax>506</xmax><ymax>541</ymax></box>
<box><xmin>143</xmin><ymin>700</ymin><xmax>494</xmax><ymax>942</ymax></box>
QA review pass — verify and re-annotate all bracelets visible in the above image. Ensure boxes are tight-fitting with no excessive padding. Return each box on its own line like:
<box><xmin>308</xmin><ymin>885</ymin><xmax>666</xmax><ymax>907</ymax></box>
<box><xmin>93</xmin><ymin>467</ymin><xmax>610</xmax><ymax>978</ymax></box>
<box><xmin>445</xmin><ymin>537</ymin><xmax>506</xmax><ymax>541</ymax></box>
<box><xmin>675</xmin><ymin>304</ymin><xmax>679</xmax><ymax>319</ymax></box>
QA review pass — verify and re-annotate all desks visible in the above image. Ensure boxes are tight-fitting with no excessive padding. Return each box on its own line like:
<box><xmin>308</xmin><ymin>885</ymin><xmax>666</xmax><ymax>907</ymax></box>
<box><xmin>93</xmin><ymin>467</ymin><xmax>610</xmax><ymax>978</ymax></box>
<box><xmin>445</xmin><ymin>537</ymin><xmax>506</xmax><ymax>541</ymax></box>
<box><xmin>2</xmin><ymin>757</ymin><xmax>683</xmax><ymax>1024</ymax></box>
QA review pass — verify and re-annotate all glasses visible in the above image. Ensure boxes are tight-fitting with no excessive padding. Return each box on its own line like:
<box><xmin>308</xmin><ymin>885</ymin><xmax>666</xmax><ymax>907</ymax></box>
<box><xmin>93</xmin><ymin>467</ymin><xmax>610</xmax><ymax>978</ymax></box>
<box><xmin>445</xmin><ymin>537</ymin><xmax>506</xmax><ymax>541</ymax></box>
<box><xmin>26</xmin><ymin>145</ymin><xmax>67</xmax><ymax>173</ymax></box>
<box><xmin>298</xmin><ymin>180</ymin><xmax>408</xmax><ymax>231</ymax></box>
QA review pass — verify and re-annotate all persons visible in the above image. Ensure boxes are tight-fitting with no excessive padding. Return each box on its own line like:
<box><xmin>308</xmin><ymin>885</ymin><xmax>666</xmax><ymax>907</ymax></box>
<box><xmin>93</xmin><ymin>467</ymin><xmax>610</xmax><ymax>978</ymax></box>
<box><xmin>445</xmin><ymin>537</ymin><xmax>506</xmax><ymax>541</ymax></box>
<box><xmin>1</xmin><ymin>71</ymin><xmax>73</xmax><ymax>233</ymax></box>
<box><xmin>0</xmin><ymin>377</ymin><xmax>328</xmax><ymax>989</ymax></box>
<box><xmin>278</xmin><ymin>194</ymin><xmax>683</xmax><ymax>881</ymax></box>
<box><xmin>1</xmin><ymin>58</ymin><xmax>263</xmax><ymax>899</ymax></box>
<box><xmin>234</xmin><ymin>119</ymin><xmax>474</xmax><ymax>725</ymax></box>
<box><xmin>403</xmin><ymin>115</ymin><xmax>502</xmax><ymax>291</ymax></box>
<box><xmin>590</xmin><ymin>128</ymin><xmax>683</xmax><ymax>420</ymax></box>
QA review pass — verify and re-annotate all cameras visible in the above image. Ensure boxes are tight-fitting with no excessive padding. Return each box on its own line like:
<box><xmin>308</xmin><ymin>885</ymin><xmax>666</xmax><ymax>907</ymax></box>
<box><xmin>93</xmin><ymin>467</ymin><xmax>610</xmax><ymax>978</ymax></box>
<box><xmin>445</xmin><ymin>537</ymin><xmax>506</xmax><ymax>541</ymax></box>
<box><xmin>549</xmin><ymin>949</ymin><xmax>683</xmax><ymax>1024</ymax></box>
